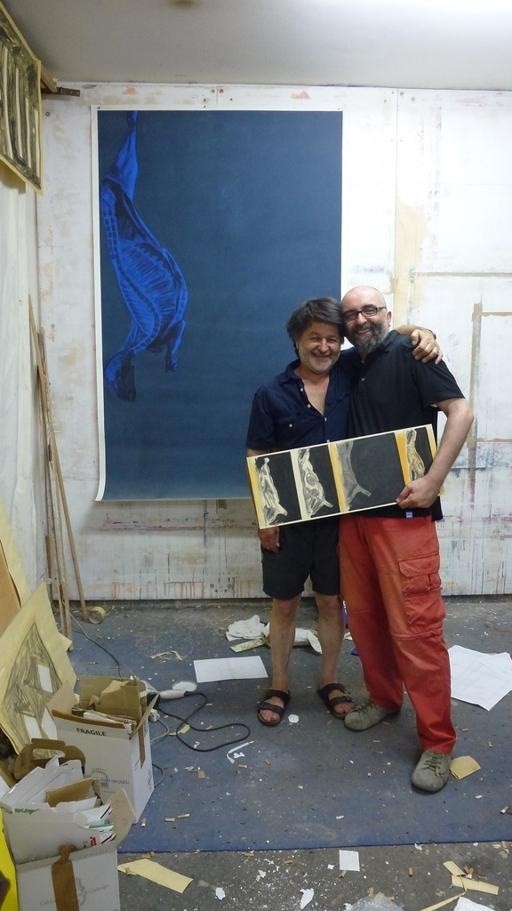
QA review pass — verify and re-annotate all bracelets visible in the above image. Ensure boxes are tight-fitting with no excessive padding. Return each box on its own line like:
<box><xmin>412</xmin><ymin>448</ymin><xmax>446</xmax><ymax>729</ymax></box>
<box><xmin>423</xmin><ymin>327</ymin><xmax>437</xmax><ymax>339</ymax></box>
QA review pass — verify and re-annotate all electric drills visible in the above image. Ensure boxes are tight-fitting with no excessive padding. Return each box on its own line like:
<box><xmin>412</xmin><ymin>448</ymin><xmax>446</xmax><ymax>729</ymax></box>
<box><xmin>131</xmin><ymin>672</ymin><xmax>193</xmax><ymax>710</ymax></box>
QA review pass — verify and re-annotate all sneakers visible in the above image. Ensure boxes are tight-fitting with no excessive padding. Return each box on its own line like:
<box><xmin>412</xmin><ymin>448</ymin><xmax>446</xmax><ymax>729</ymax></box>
<box><xmin>410</xmin><ymin>747</ymin><xmax>451</xmax><ymax>796</ymax></box>
<box><xmin>344</xmin><ymin>698</ymin><xmax>402</xmax><ymax>733</ymax></box>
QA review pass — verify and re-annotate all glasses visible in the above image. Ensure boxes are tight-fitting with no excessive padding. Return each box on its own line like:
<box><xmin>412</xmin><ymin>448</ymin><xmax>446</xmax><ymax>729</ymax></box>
<box><xmin>344</xmin><ymin>305</ymin><xmax>389</xmax><ymax>320</ymax></box>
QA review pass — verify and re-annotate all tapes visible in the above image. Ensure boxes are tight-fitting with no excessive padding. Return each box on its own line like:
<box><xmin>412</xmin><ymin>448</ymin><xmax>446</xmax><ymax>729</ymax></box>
<box><xmin>87</xmin><ymin>606</ymin><xmax>105</xmax><ymax>624</ymax></box>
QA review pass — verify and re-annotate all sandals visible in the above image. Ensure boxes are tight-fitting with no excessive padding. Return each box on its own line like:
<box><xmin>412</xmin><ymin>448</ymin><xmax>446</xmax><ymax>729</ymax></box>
<box><xmin>316</xmin><ymin>681</ymin><xmax>356</xmax><ymax>722</ymax></box>
<box><xmin>256</xmin><ymin>686</ymin><xmax>293</xmax><ymax>727</ymax></box>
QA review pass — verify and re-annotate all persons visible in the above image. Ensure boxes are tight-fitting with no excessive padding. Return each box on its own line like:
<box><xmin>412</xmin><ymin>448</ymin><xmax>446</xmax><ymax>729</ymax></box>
<box><xmin>339</xmin><ymin>283</ymin><xmax>477</xmax><ymax>797</ymax></box>
<box><xmin>245</xmin><ymin>297</ymin><xmax>442</xmax><ymax>730</ymax></box>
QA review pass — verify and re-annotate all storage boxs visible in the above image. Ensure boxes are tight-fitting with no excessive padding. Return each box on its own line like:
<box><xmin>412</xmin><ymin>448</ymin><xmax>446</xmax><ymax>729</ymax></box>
<box><xmin>1</xmin><ymin>731</ymin><xmax>144</xmax><ymax>911</ymax></box>
<box><xmin>48</xmin><ymin>676</ymin><xmax>162</xmax><ymax>825</ymax></box>
